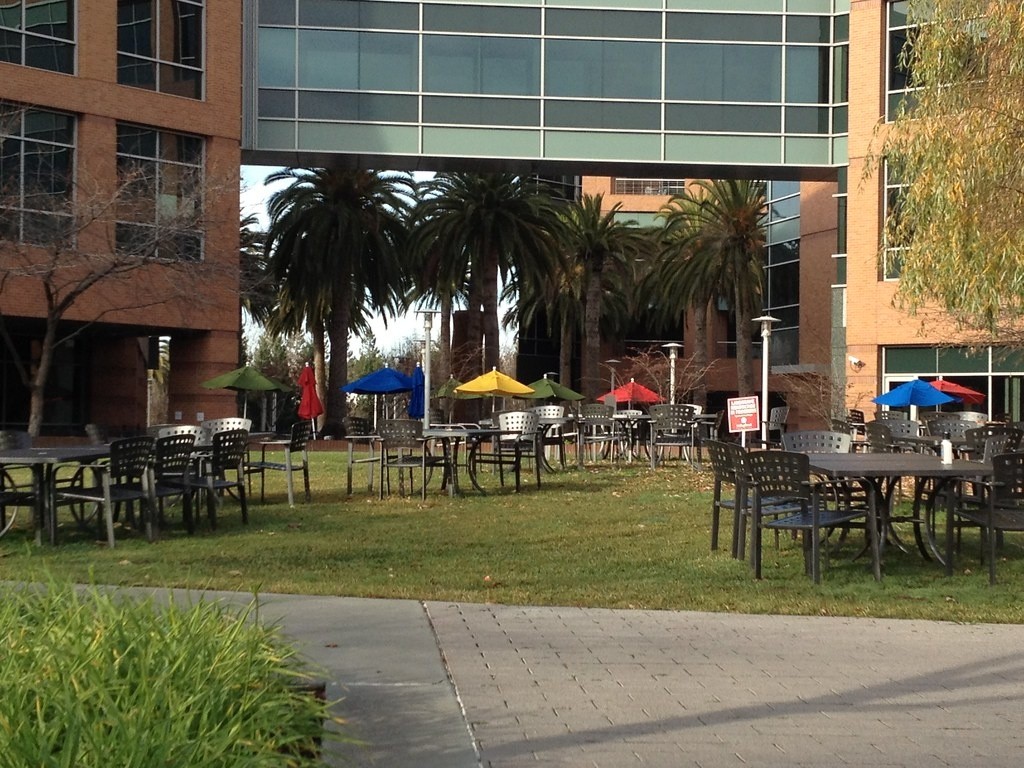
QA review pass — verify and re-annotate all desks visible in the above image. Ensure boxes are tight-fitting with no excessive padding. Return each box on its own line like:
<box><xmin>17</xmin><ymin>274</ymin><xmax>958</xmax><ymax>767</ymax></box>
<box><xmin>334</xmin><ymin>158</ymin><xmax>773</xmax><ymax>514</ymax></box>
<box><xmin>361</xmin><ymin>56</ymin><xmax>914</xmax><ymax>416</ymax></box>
<box><xmin>893</xmin><ymin>435</ymin><xmax>987</xmax><ymax>447</ymax></box>
<box><xmin>0</xmin><ymin>443</ymin><xmax>213</xmax><ymax>546</ymax></box>
<box><xmin>423</xmin><ymin>429</ymin><xmax>522</xmax><ymax>498</ymax></box>
<box><xmin>614</xmin><ymin>414</ymin><xmax>651</xmax><ymax>464</ymax></box>
<box><xmin>806</xmin><ymin>452</ymin><xmax>993</xmax><ymax>583</ymax></box>
<box><xmin>538</xmin><ymin>418</ymin><xmax>573</xmax><ymax>473</ymax></box>
<box><xmin>694</xmin><ymin>413</ymin><xmax>717</xmax><ymax>472</ymax></box>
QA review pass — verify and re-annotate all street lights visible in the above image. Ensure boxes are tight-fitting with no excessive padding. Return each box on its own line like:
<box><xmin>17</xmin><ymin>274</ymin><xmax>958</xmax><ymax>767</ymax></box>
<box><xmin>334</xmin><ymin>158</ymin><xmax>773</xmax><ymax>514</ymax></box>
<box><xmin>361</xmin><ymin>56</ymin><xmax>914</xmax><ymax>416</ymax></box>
<box><xmin>414</xmin><ymin>308</ymin><xmax>442</xmax><ymax>429</ymax></box>
<box><xmin>605</xmin><ymin>359</ymin><xmax>621</xmax><ymax>391</ymax></box>
<box><xmin>751</xmin><ymin>315</ymin><xmax>782</xmax><ymax>449</ymax></box>
<box><xmin>661</xmin><ymin>343</ymin><xmax>684</xmax><ymax>433</ymax></box>
<box><xmin>413</xmin><ymin>338</ymin><xmax>434</xmax><ymax>373</ymax></box>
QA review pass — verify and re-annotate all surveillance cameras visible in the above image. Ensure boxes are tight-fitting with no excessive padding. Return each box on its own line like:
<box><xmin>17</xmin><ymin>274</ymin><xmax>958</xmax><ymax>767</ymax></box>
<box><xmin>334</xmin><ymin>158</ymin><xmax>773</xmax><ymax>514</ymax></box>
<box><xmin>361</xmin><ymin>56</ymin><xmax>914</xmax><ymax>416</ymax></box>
<box><xmin>848</xmin><ymin>356</ymin><xmax>861</xmax><ymax>366</ymax></box>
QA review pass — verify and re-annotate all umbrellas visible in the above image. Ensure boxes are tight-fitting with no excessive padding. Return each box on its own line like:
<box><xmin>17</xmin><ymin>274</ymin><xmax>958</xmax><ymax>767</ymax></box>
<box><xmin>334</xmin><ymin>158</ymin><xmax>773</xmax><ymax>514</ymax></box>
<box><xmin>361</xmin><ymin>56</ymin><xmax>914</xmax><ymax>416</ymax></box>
<box><xmin>515</xmin><ymin>374</ymin><xmax>585</xmax><ymax>461</ymax></box>
<box><xmin>297</xmin><ymin>362</ymin><xmax>324</xmax><ymax>440</ymax></box>
<box><xmin>431</xmin><ymin>374</ymin><xmax>489</xmax><ymax>429</ymax></box>
<box><xmin>596</xmin><ymin>378</ymin><xmax>665</xmax><ymax>409</ymax></box>
<box><xmin>201</xmin><ymin>365</ymin><xmax>290</xmax><ymax>420</ymax></box>
<box><xmin>340</xmin><ymin>368</ymin><xmax>411</xmax><ymax>433</ymax></box>
<box><xmin>928</xmin><ymin>376</ymin><xmax>985</xmax><ymax>418</ymax></box>
<box><xmin>871</xmin><ymin>377</ymin><xmax>954</xmax><ymax>433</ymax></box>
<box><xmin>407</xmin><ymin>362</ymin><xmax>426</xmax><ymax>437</ymax></box>
<box><xmin>453</xmin><ymin>365</ymin><xmax>536</xmax><ymax>474</ymax></box>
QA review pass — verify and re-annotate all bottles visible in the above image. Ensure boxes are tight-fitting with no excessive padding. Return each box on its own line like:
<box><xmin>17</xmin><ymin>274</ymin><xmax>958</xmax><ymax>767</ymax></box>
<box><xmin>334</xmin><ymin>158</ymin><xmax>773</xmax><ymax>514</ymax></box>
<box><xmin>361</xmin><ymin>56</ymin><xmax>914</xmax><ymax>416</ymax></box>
<box><xmin>940</xmin><ymin>440</ymin><xmax>955</xmax><ymax>465</ymax></box>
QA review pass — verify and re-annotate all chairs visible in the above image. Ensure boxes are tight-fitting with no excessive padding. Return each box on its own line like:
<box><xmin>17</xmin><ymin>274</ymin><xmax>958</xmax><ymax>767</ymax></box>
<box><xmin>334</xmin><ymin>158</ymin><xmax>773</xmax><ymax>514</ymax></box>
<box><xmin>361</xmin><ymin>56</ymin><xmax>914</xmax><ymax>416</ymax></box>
<box><xmin>49</xmin><ymin>416</ymin><xmax>312</xmax><ymax>549</ymax></box>
<box><xmin>342</xmin><ymin>417</ymin><xmax>443</xmax><ymax>499</ymax></box>
<box><xmin>492</xmin><ymin>403</ymin><xmax>1024</xmax><ymax>584</ymax></box>
